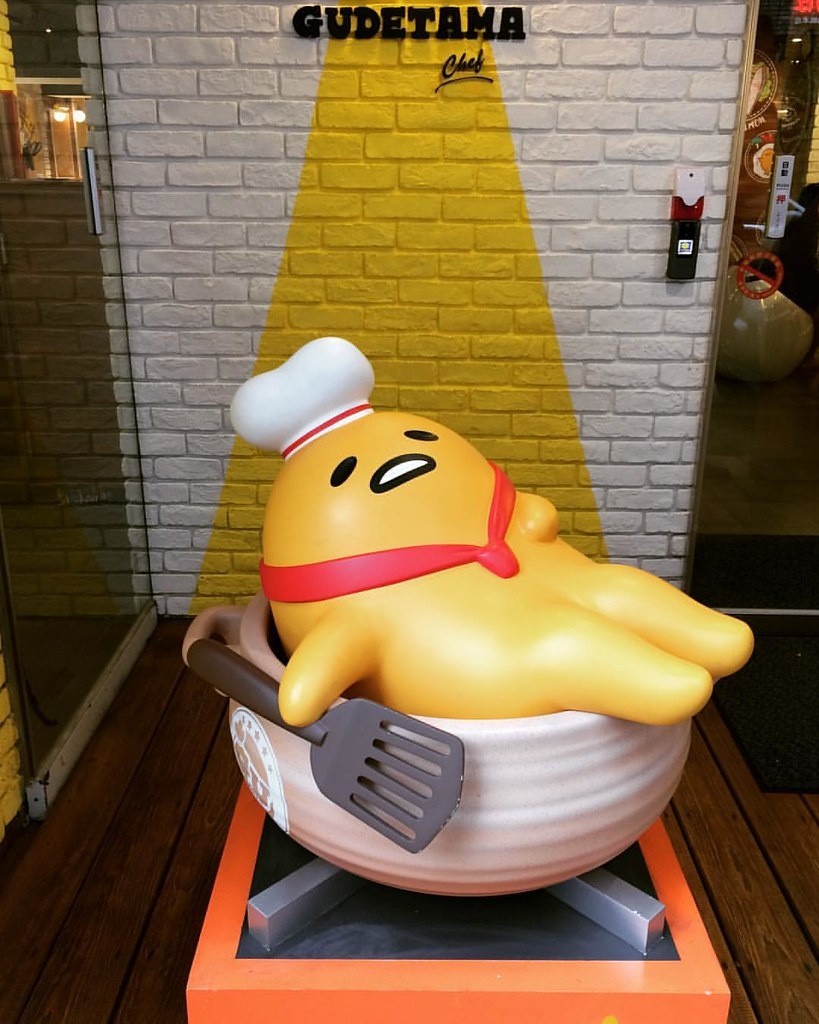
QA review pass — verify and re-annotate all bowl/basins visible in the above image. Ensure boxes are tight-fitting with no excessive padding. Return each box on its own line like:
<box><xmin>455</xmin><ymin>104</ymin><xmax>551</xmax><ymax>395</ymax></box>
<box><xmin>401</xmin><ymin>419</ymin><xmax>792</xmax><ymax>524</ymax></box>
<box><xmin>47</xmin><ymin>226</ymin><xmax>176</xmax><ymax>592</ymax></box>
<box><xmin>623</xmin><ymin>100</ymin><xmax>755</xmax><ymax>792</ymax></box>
<box><xmin>180</xmin><ymin>592</ymin><xmax>695</xmax><ymax>895</ymax></box>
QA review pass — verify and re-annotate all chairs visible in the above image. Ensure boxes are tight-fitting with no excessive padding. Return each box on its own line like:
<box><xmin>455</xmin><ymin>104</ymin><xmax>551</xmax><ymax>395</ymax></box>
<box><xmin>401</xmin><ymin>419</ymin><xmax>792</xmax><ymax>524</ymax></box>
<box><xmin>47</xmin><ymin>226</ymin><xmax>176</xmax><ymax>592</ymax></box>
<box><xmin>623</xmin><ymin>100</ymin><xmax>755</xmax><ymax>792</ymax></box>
<box><xmin>713</xmin><ymin>264</ymin><xmax>815</xmax><ymax>380</ymax></box>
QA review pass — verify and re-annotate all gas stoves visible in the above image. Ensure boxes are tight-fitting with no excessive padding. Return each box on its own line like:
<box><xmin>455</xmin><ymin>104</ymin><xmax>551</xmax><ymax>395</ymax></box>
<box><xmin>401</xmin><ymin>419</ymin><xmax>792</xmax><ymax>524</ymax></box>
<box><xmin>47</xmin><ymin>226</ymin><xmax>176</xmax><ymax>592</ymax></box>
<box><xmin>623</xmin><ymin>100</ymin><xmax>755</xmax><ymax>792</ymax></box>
<box><xmin>184</xmin><ymin>781</ymin><xmax>732</xmax><ymax>1024</ymax></box>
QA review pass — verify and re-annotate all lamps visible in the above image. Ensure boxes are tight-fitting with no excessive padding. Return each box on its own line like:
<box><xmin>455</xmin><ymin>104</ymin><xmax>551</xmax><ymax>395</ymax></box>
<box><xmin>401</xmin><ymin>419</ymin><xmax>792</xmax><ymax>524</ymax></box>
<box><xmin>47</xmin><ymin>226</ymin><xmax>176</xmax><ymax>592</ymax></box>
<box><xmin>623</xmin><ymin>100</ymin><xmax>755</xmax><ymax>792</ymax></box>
<box><xmin>73</xmin><ymin>102</ymin><xmax>85</xmax><ymax>123</ymax></box>
